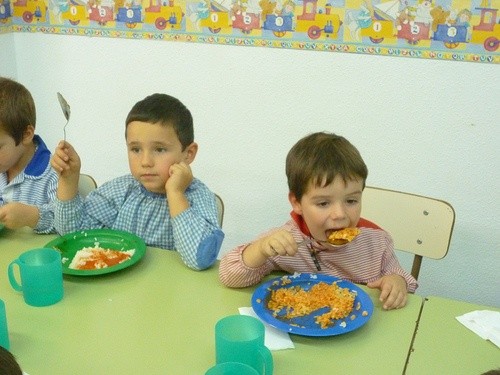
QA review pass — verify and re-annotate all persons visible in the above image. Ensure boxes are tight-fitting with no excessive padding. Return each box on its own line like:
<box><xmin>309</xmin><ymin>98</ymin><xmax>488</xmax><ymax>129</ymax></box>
<box><xmin>50</xmin><ymin>93</ymin><xmax>224</xmax><ymax>271</ymax></box>
<box><xmin>0</xmin><ymin>76</ymin><xmax>62</xmax><ymax>236</ymax></box>
<box><xmin>218</xmin><ymin>134</ymin><xmax>418</xmax><ymax>310</ymax></box>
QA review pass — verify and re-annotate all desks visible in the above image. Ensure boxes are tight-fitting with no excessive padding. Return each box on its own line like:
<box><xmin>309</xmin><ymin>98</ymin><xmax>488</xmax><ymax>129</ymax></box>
<box><xmin>0</xmin><ymin>226</ymin><xmax>424</xmax><ymax>375</ymax></box>
<box><xmin>403</xmin><ymin>293</ymin><xmax>500</xmax><ymax>375</ymax></box>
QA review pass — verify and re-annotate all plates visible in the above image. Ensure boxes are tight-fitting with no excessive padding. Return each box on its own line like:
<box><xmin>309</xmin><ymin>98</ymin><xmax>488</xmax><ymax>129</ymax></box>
<box><xmin>250</xmin><ymin>273</ymin><xmax>373</xmax><ymax>336</ymax></box>
<box><xmin>43</xmin><ymin>228</ymin><xmax>146</xmax><ymax>276</ymax></box>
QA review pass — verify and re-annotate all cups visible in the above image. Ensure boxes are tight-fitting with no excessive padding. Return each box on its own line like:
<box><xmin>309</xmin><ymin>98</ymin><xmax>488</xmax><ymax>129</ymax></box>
<box><xmin>0</xmin><ymin>300</ymin><xmax>10</xmax><ymax>351</ymax></box>
<box><xmin>8</xmin><ymin>247</ymin><xmax>64</xmax><ymax>306</ymax></box>
<box><xmin>215</xmin><ymin>314</ymin><xmax>273</xmax><ymax>375</ymax></box>
<box><xmin>204</xmin><ymin>362</ymin><xmax>259</xmax><ymax>375</ymax></box>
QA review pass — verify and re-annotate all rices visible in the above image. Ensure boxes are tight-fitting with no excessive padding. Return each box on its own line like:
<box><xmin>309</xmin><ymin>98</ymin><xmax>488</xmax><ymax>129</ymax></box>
<box><xmin>62</xmin><ymin>242</ymin><xmax>135</xmax><ymax>270</ymax></box>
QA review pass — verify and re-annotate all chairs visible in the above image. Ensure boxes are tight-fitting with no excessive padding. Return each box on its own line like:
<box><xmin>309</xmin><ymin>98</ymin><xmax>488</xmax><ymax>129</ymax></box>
<box><xmin>356</xmin><ymin>182</ymin><xmax>456</xmax><ymax>282</ymax></box>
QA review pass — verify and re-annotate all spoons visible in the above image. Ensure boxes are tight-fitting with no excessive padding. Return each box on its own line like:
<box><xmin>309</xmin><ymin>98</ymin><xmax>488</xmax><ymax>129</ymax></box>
<box><xmin>57</xmin><ymin>92</ymin><xmax>71</xmax><ymax>142</ymax></box>
<box><xmin>296</xmin><ymin>239</ymin><xmax>349</xmax><ymax>248</ymax></box>
